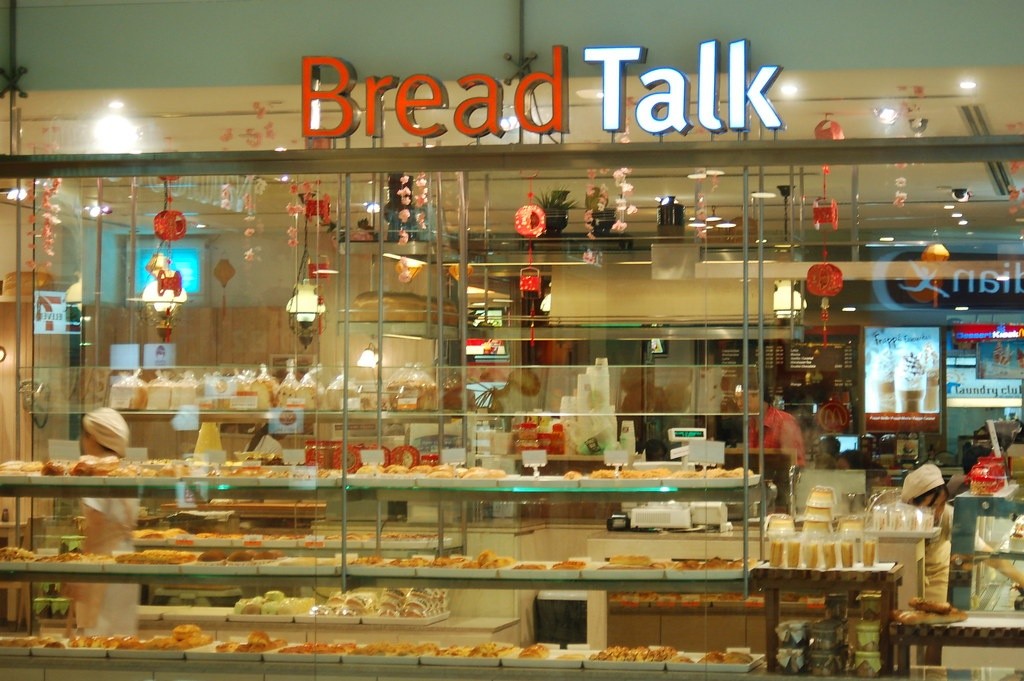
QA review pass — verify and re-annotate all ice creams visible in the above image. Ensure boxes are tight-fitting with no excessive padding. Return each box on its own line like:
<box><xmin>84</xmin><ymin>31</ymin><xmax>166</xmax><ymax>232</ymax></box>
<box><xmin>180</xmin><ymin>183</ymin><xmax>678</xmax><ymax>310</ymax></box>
<box><xmin>993</xmin><ymin>341</ymin><xmax>1024</xmax><ymax>375</ymax></box>
<box><xmin>872</xmin><ymin>343</ymin><xmax>938</xmax><ymax>413</ymax></box>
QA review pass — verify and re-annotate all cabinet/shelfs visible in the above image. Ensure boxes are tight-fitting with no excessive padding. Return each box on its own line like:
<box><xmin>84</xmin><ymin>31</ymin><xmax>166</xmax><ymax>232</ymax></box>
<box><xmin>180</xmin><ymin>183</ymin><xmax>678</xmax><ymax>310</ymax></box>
<box><xmin>0</xmin><ymin>367</ymin><xmax>768</xmax><ymax>681</ymax></box>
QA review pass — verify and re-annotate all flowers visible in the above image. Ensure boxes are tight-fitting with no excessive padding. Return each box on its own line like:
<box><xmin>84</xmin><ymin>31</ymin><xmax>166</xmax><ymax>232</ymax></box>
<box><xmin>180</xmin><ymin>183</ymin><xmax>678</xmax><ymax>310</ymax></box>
<box><xmin>692</xmin><ymin>126</ymin><xmax>720</xmax><ymax>239</ymax></box>
<box><xmin>287</xmin><ymin>174</ymin><xmax>331</xmax><ymax>247</ymax></box>
<box><xmin>395</xmin><ymin>171</ymin><xmax>429</xmax><ymax>281</ymax></box>
<box><xmin>612</xmin><ymin>97</ymin><xmax>636</xmax><ymax>231</ymax></box>
<box><xmin>25</xmin><ymin>178</ymin><xmax>62</xmax><ymax>272</ymax></box>
<box><xmin>892</xmin><ymin>163</ymin><xmax>908</xmax><ymax>208</ymax></box>
<box><xmin>580</xmin><ymin>140</ymin><xmax>610</xmax><ymax>268</ymax></box>
<box><xmin>220</xmin><ymin>175</ymin><xmax>270</xmax><ymax>261</ymax></box>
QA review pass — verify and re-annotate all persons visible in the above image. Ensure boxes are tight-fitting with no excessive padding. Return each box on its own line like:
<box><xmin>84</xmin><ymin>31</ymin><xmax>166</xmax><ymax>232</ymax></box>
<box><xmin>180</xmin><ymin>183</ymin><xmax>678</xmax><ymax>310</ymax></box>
<box><xmin>809</xmin><ymin>436</ymin><xmax>888</xmax><ymax>514</ymax></box>
<box><xmin>644</xmin><ymin>438</ymin><xmax>668</xmax><ymax>460</ymax></box>
<box><xmin>619</xmin><ymin>367</ymin><xmax>676</xmax><ymax>460</ymax></box>
<box><xmin>243</xmin><ymin>422</ymin><xmax>287</xmax><ymax>528</ymax></box>
<box><xmin>902</xmin><ymin>463</ymin><xmax>1024</xmax><ymax>604</ymax></box>
<box><xmin>733</xmin><ymin>373</ymin><xmax>805</xmax><ymax>471</ymax></box>
<box><xmin>59</xmin><ymin>405</ymin><xmax>140</xmax><ymax>638</ymax></box>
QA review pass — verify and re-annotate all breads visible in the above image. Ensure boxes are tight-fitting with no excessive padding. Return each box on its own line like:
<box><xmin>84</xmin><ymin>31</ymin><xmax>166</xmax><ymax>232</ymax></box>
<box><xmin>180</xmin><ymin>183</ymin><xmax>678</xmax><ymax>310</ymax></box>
<box><xmin>892</xmin><ymin>597</ymin><xmax>967</xmax><ymax>626</ymax></box>
<box><xmin>107</xmin><ymin>374</ymin><xmax>438</xmax><ymax>411</ymax></box>
<box><xmin>768</xmin><ymin>490</ymin><xmax>875</xmax><ymax>569</ymax></box>
<box><xmin>0</xmin><ymin>464</ymin><xmax>752</xmax><ymax>665</ymax></box>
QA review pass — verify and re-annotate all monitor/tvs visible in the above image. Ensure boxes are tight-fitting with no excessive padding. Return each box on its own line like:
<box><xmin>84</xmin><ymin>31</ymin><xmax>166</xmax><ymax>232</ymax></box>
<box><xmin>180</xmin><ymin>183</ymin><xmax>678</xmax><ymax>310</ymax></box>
<box><xmin>127</xmin><ymin>239</ymin><xmax>208</xmax><ymax>303</ymax></box>
<box><xmin>819</xmin><ymin>434</ymin><xmax>860</xmax><ymax>454</ymax></box>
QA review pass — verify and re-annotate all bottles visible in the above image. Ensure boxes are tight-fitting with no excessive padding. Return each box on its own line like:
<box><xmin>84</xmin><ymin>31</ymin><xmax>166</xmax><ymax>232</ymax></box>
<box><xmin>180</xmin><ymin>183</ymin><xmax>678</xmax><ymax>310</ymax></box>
<box><xmin>657</xmin><ymin>196</ymin><xmax>685</xmax><ymax>236</ymax></box>
<box><xmin>970</xmin><ymin>437</ymin><xmax>1024</xmax><ymax>504</ymax></box>
<box><xmin>730</xmin><ymin>205</ymin><xmax>757</xmax><ymax>242</ymax></box>
<box><xmin>705</xmin><ymin>204</ymin><xmax>736</xmax><ymax>243</ymax></box>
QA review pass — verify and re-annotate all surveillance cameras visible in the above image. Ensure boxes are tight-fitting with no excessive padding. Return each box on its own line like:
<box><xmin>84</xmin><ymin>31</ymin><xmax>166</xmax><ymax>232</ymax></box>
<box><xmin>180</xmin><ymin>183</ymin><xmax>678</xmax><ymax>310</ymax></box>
<box><xmin>951</xmin><ymin>189</ymin><xmax>967</xmax><ymax>199</ymax></box>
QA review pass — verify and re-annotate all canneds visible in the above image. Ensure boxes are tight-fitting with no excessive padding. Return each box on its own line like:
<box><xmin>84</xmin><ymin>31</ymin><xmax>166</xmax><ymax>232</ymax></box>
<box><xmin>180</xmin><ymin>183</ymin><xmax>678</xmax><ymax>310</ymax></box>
<box><xmin>304</xmin><ymin>439</ymin><xmax>440</xmax><ymax>469</ymax></box>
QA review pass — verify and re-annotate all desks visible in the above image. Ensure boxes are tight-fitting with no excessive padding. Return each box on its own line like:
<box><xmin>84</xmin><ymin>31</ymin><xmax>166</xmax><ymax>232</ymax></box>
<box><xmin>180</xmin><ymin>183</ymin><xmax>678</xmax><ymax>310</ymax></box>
<box><xmin>889</xmin><ymin>611</ymin><xmax>1024</xmax><ymax>667</ymax></box>
<box><xmin>750</xmin><ymin>560</ymin><xmax>904</xmax><ymax>677</ymax></box>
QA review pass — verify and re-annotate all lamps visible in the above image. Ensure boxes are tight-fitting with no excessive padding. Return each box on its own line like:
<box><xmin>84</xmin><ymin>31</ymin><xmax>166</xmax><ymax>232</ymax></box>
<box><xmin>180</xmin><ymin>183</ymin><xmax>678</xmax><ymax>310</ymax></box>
<box><xmin>909</xmin><ymin>119</ymin><xmax>928</xmax><ymax>134</ymax></box>
<box><xmin>139</xmin><ymin>177</ymin><xmax>188</xmax><ymax>342</ymax></box>
<box><xmin>875</xmin><ymin>103</ymin><xmax>899</xmax><ymax>124</ymax></box>
<box><xmin>356</xmin><ymin>342</ymin><xmax>380</xmax><ymax>368</ymax></box>
<box><xmin>285</xmin><ymin>193</ymin><xmax>327</xmax><ymax>349</ymax></box>
<box><xmin>773</xmin><ymin>186</ymin><xmax>808</xmax><ymax>317</ymax></box>
<box><xmin>687</xmin><ymin>204</ymin><xmax>736</xmax><ymax>229</ymax></box>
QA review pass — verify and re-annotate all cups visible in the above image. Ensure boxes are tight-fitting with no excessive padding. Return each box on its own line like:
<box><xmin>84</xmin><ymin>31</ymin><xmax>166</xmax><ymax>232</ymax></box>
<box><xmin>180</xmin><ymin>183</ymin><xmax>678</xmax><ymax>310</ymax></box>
<box><xmin>856</xmin><ymin>623</ymin><xmax>880</xmax><ymax>651</ymax></box>
<box><xmin>854</xmin><ymin>651</ymin><xmax>881</xmax><ymax>678</ymax></box>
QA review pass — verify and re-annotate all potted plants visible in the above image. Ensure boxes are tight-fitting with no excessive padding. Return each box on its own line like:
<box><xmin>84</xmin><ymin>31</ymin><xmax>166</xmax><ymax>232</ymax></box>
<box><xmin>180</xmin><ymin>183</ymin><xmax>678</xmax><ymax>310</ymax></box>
<box><xmin>584</xmin><ymin>188</ymin><xmax>617</xmax><ymax>235</ymax></box>
<box><xmin>533</xmin><ymin>183</ymin><xmax>579</xmax><ymax>235</ymax></box>
<box><xmin>657</xmin><ymin>202</ymin><xmax>685</xmax><ymax>242</ymax></box>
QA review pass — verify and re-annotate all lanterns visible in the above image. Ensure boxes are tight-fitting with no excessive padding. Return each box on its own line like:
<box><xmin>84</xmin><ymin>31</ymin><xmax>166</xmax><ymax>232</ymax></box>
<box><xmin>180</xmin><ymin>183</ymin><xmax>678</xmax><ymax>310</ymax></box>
<box><xmin>153</xmin><ymin>210</ymin><xmax>186</xmax><ymax>345</ymax></box>
<box><xmin>806</xmin><ymin>119</ymin><xmax>846</xmax><ymax>349</ymax></box>
<box><xmin>306</xmin><ymin>192</ymin><xmax>331</xmax><ymax>335</ymax></box>
<box><xmin>515</xmin><ymin>205</ymin><xmax>547</xmax><ymax>345</ymax></box>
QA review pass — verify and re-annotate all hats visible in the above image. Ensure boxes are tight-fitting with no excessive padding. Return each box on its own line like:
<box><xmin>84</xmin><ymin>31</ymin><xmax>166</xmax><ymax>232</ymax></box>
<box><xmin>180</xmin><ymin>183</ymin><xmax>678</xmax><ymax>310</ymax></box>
<box><xmin>82</xmin><ymin>407</ymin><xmax>129</xmax><ymax>458</ymax></box>
<box><xmin>901</xmin><ymin>463</ymin><xmax>945</xmax><ymax>504</ymax></box>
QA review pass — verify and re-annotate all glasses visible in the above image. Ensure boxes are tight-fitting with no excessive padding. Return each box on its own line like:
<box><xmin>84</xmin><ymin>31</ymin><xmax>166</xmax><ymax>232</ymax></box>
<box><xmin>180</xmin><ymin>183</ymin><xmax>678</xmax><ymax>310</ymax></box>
<box><xmin>732</xmin><ymin>396</ymin><xmax>744</xmax><ymax>404</ymax></box>
